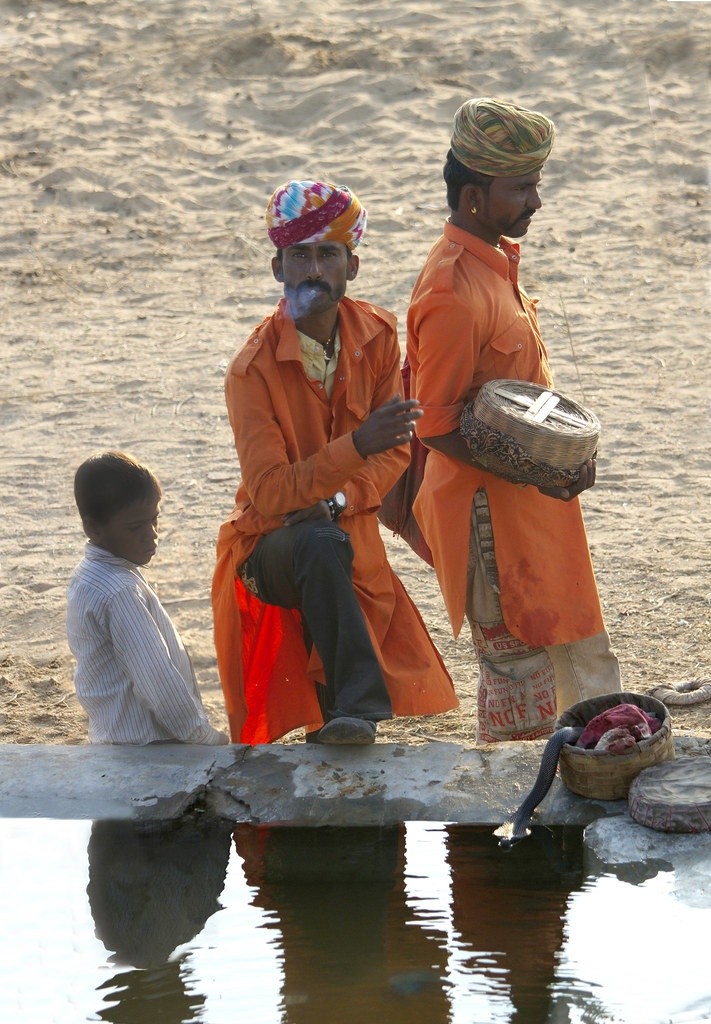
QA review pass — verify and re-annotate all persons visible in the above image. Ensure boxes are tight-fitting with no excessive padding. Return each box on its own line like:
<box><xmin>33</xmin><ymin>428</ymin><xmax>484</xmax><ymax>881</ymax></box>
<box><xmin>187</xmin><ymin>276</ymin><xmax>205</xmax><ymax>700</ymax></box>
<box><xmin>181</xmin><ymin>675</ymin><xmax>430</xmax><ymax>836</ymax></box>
<box><xmin>225</xmin><ymin>181</ymin><xmax>461</xmax><ymax>746</ymax></box>
<box><xmin>67</xmin><ymin>451</ymin><xmax>230</xmax><ymax>747</ymax></box>
<box><xmin>407</xmin><ymin>97</ymin><xmax>623</xmax><ymax>744</ymax></box>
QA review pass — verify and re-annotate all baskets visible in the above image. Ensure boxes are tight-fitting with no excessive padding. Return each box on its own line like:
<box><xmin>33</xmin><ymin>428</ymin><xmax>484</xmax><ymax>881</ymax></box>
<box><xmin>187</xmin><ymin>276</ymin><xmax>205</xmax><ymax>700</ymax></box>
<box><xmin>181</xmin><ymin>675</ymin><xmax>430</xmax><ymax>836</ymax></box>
<box><xmin>551</xmin><ymin>692</ymin><xmax>676</xmax><ymax>801</ymax></box>
<box><xmin>460</xmin><ymin>378</ymin><xmax>601</xmax><ymax>488</ymax></box>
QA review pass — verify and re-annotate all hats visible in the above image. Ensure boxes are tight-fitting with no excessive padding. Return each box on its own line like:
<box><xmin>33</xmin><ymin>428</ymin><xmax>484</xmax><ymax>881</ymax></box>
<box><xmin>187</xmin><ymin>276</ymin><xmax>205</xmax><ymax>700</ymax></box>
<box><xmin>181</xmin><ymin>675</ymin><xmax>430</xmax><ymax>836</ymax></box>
<box><xmin>451</xmin><ymin>97</ymin><xmax>557</xmax><ymax>177</ymax></box>
<box><xmin>265</xmin><ymin>179</ymin><xmax>366</xmax><ymax>249</ymax></box>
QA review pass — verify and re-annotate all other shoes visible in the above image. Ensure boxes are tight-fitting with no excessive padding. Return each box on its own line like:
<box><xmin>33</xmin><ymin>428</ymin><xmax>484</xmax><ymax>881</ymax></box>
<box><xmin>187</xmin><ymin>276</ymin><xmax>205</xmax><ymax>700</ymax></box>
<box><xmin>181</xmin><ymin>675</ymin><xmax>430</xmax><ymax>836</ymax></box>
<box><xmin>301</xmin><ymin>709</ymin><xmax>376</xmax><ymax>746</ymax></box>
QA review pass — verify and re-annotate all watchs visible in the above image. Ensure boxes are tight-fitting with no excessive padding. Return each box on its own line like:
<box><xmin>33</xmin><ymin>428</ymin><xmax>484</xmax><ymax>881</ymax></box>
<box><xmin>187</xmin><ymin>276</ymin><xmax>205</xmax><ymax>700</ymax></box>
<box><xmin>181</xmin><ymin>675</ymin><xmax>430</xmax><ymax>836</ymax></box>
<box><xmin>332</xmin><ymin>491</ymin><xmax>347</xmax><ymax>517</ymax></box>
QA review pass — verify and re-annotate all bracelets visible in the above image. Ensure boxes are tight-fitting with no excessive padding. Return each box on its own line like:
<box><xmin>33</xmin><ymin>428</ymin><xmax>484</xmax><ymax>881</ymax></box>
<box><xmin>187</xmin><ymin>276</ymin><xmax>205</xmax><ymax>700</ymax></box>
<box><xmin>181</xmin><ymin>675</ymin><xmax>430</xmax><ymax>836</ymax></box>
<box><xmin>325</xmin><ymin>499</ymin><xmax>337</xmax><ymax>521</ymax></box>
